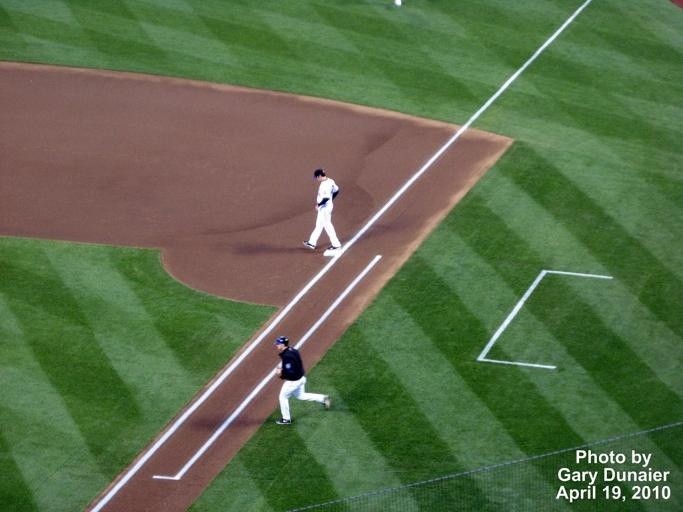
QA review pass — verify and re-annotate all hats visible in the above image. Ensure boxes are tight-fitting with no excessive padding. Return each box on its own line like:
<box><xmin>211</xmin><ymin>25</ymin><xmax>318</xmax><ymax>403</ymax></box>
<box><xmin>313</xmin><ymin>170</ymin><xmax>321</xmax><ymax>178</ymax></box>
<box><xmin>273</xmin><ymin>337</ymin><xmax>288</xmax><ymax>345</ymax></box>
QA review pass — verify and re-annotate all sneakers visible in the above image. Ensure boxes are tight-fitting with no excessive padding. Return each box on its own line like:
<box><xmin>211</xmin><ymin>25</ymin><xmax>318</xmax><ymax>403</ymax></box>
<box><xmin>302</xmin><ymin>241</ymin><xmax>316</xmax><ymax>249</ymax></box>
<box><xmin>324</xmin><ymin>397</ymin><xmax>330</xmax><ymax>411</ymax></box>
<box><xmin>276</xmin><ymin>419</ymin><xmax>291</xmax><ymax>425</ymax></box>
<box><xmin>327</xmin><ymin>246</ymin><xmax>340</xmax><ymax>250</ymax></box>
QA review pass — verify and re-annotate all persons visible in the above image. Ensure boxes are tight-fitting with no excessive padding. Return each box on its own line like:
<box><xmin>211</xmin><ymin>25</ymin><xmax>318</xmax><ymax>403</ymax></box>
<box><xmin>273</xmin><ymin>335</ymin><xmax>330</xmax><ymax>424</ymax></box>
<box><xmin>302</xmin><ymin>169</ymin><xmax>341</xmax><ymax>250</ymax></box>
